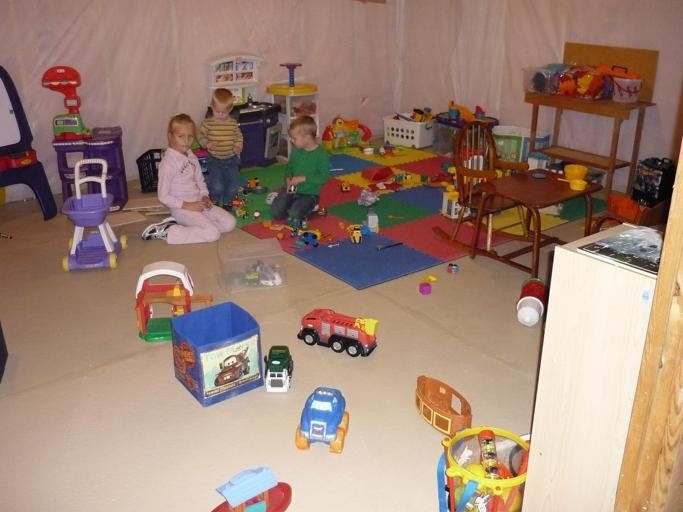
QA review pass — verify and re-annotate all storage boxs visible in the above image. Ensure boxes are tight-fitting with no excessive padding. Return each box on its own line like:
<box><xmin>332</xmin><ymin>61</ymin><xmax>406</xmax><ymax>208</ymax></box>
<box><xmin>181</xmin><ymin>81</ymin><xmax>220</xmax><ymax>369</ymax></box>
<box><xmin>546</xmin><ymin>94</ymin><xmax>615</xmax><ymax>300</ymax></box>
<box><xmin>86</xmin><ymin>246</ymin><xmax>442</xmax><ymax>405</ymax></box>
<box><xmin>215</xmin><ymin>235</ymin><xmax>288</xmax><ymax>297</ymax></box>
<box><xmin>521</xmin><ymin>60</ymin><xmax>574</xmax><ymax>96</ymax></box>
<box><xmin>433</xmin><ymin>110</ymin><xmax>501</xmax><ymax>154</ymax></box>
<box><xmin>168</xmin><ymin>301</ymin><xmax>264</xmax><ymax>408</ymax></box>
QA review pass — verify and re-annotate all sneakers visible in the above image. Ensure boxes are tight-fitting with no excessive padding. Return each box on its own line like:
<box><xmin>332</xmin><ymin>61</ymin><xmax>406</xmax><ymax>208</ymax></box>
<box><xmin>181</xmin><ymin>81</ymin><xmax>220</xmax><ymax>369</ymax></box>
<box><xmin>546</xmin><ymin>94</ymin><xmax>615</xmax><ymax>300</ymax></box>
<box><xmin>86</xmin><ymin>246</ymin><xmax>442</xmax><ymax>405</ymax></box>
<box><xmin>142</xmin><ymin>217</ymin><xmax>176</xmax><ymax>240</ymax></box>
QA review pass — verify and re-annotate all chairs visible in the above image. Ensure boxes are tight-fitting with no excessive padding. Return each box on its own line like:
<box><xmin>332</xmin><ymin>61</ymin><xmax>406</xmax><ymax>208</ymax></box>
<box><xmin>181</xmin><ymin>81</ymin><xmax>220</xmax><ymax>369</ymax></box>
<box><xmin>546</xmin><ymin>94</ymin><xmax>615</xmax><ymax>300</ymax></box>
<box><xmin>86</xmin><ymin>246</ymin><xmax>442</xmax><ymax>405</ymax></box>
<box><xmin>427</xmin><ymin>119</ymin><xmax>538</xmax><ymax>258</ymax></box>
<box><xmin>590</xmin><ymin>202</ymin><xmax>672</xmax><ymax>250</ymax></box>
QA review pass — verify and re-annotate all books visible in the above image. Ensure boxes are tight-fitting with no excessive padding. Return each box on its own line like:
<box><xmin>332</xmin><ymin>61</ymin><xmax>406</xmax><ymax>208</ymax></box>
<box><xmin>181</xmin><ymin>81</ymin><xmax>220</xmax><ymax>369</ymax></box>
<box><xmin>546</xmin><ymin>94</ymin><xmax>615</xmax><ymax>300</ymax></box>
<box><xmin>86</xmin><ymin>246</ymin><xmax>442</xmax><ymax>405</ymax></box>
<box><xmin>576</xmin><ymin>224</ymin><xmax>665</xmax><ymax>279</ymax></box>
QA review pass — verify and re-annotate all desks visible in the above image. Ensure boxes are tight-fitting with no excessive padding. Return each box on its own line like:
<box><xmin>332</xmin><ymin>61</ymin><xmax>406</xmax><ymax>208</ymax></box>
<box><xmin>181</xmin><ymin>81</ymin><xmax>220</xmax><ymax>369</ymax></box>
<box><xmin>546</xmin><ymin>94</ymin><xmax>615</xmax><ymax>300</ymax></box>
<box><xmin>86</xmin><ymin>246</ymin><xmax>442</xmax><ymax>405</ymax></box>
<box><xmin>467</xmin><ymin>167</ymin><xmax>603</xmax><ymax>276</ymax></box>
<box><xmin>212</xmin><ymin>102</ymin><xmax>279</xmax><ymax>166</ymax></box>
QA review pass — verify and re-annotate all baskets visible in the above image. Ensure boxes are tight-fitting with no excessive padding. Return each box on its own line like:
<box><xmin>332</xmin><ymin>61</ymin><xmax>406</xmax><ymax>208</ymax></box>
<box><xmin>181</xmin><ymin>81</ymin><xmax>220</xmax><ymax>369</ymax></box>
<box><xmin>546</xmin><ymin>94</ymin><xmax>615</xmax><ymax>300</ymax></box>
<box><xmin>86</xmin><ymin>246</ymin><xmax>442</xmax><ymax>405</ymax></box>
<box><xmin>137</xmin><ymin>149</ymin><xmax>161</xmax><ymax>192</ymax></box>
<box><xmin>383</xmin><ymin>114</ymin><xmax>432</xmax><ymax>149</ymax></box>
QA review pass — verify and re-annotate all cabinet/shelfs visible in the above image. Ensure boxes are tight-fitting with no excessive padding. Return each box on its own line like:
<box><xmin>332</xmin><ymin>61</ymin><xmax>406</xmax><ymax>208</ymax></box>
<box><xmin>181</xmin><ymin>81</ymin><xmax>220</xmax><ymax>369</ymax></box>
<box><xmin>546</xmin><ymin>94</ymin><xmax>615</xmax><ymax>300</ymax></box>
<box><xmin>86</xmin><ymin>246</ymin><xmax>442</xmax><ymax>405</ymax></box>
<box><xmin>51</xmin><ymin>127</ymin><xmax>126</xmax><ymax>213</ymax></box>
<box><xmin>514</xmin><ymin>223</ymin><xmax>679</xmax><ymax>512</ymax></box>
<box><xmin>269</xmin><ymin>81</ymin><xmax>322</xmax><ymax>165</ymax></box>
<box><xmin>520</xmin><ymin>40</ymin><xmax>659</xmax><ymax>203</ymax></box>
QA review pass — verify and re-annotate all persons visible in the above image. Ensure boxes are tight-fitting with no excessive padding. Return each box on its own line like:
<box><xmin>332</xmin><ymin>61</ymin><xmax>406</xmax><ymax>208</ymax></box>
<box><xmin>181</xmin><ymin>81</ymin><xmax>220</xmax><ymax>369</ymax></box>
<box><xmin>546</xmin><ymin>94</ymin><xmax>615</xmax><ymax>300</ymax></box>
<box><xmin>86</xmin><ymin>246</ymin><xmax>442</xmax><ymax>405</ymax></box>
<box><xmin>270</xmin><ymin>116</ymin><xmax>330</xmax><ymax>219</ymax></box>
<box><xmin>197</xmin><ymin>88</ymin><xmax>243</xmax><ymax>211</ymax></box>
<box><xmin>142</xmin><ymin>114</ymin><xmax>236</xmax><ymax>245</ymax></box>
